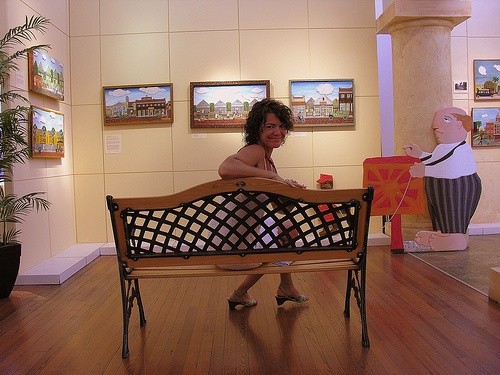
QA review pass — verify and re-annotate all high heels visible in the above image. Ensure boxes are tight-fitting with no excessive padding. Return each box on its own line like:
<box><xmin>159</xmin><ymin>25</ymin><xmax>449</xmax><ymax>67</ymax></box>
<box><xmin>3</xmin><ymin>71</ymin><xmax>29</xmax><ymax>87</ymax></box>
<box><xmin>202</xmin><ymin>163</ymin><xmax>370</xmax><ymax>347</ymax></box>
<box><xmin>226</xmin><ymin>289</ymin><xmax>257</xmax><ymax>309</ymax></box>
<box><xmin>275</xmin><ymin>285</ymin><xmax>309</xmax><ymax>305</ymax></box>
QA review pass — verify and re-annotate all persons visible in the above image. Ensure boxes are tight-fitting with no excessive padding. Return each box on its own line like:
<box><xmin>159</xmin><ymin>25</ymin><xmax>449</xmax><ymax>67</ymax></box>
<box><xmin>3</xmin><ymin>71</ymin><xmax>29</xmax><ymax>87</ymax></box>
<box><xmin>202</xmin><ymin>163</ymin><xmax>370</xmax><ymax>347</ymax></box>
<box><xmin>217</xmin><ymin>97</ymin><xmax>311</xmax><ymax>311</ymax></box>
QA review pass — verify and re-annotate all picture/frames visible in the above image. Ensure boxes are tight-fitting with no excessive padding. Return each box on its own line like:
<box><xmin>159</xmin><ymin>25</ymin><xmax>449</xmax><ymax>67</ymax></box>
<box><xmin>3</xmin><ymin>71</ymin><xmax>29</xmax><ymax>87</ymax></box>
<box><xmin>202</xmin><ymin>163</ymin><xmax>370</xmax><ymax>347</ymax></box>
<box><xmin>472</xmin><ymin>59</ymin><xmax>500</xmax><ymax>100</ymax></box>
<box><xmin>101</xmin><ymin>82</ymin><xmax>174</xmax><ymax>125</ymax></box>
<box><xmin>471</xmin><ymin>107</ymin><xmax>500</xmax><ymax>147</ymax></box>
<box><xmin>29</xmin><ymin>105</ymin><xmax>65</xmax><ymax>159</ymax></box>
<box><xmin>287</xmin><ymin>79</ymin><xmax>357</xmax><ymax>128</ymax></box>
<box><xmin>189</xmin><ymin>80</ymin><xmax>270</xmax><ymax>128</ymax></box>
<box><xmin>28</xmin><ymin>48</ymin><xmax>65</xmax><ymax>102</ymax></box>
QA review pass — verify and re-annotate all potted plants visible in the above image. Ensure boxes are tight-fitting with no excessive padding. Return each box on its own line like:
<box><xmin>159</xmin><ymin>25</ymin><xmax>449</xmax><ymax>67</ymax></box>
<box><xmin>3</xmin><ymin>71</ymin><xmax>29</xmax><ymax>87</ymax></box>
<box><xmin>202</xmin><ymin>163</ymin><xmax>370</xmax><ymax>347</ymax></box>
<box><xmin>0</xmin><ymin>14</ymin><xmax>54</xmax><ymax>301</ymax></box>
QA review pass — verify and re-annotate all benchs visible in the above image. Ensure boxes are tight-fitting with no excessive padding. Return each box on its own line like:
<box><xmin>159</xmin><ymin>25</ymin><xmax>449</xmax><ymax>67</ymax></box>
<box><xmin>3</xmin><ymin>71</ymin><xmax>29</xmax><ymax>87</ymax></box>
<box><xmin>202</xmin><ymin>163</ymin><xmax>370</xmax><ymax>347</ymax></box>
<box><xmin>103</xmin><ymin>177</ymin><xmax>374</xmax><ymax>359</ymax></box>
